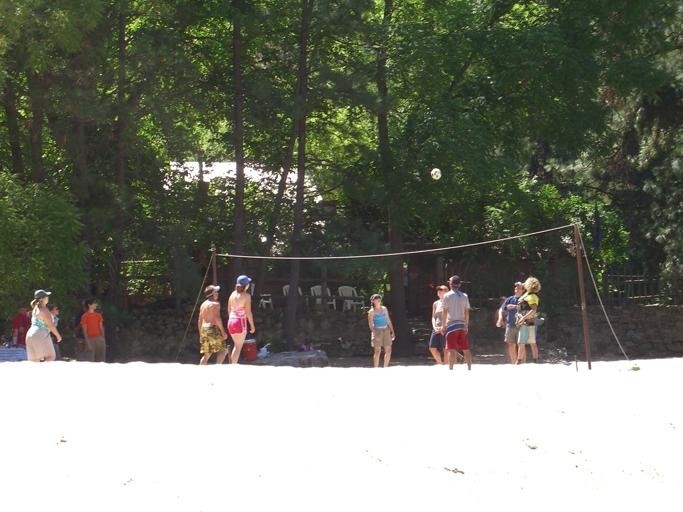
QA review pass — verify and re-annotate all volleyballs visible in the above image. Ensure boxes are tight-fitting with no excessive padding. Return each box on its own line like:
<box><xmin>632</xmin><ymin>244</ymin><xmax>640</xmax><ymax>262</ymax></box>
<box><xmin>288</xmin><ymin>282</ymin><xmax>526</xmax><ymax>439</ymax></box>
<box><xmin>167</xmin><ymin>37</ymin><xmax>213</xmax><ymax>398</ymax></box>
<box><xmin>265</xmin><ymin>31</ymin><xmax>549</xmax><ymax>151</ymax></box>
<box><xmin>431</xmin><ymin>168</ymin><xmax>442</xmax><ymax>179</ymax></box>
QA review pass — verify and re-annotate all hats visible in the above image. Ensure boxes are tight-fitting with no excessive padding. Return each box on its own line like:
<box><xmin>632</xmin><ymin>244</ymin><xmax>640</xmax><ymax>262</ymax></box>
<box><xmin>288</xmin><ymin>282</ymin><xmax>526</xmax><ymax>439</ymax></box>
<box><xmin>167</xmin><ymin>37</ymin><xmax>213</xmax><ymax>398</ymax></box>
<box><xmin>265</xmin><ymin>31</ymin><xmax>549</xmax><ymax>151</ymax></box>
<box><xmin>514</xmin><ymin>281</ymin><xmax>524</xmax><ymax>289</ymax></box>
<box><xmin>435</xmin><ymin>285</ymin><xmax>449</xmax><ymax>291</ymax></box>
<box><xmin>34</xmin><ymin>289</ymin><xmax>52</xmax><ymax>299</ymax></box>
<box><xmin>236</xmin><ymin>275</ymin><xmax>252</xmax><ymax>286</ymax></box>
<box><xmin>450</xmin><ymin>274</ymin><xmax>461</xmax><ymax>286</ymax></box>
<box><xmin>204</xmin><ymin>285</ymin><xmax>221</xmax><ymax>298</ymax></box>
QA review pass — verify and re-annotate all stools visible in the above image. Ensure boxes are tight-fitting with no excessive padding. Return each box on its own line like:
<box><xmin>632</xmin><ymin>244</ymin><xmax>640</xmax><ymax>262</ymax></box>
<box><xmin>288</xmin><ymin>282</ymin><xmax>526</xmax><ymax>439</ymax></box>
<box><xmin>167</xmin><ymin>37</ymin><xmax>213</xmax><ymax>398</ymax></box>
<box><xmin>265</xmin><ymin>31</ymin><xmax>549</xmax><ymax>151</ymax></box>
<box><xmin>257</xmin><ymin>292</ymin><xmax>274</xmax><ymax>309</ymax></box>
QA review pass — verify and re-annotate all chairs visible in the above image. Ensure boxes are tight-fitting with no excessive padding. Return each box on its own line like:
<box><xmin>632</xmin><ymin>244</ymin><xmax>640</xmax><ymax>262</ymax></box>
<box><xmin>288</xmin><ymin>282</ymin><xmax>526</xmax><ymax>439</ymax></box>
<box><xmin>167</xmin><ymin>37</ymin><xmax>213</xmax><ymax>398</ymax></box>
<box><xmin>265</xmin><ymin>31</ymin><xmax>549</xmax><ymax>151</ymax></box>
<box><xmin>337</xmin><ymin>286</ymin><xmax>365</xmax><ymax>312</ymax></box>
<box><xmin>282</xmin><ymin>285</ymin><xmax>308</xmax><ymax>307</ymax></box>
<box><xmin>310</xmin><ymin>285</ymin><xmax>336</xmax><ymax>310</ymax></box>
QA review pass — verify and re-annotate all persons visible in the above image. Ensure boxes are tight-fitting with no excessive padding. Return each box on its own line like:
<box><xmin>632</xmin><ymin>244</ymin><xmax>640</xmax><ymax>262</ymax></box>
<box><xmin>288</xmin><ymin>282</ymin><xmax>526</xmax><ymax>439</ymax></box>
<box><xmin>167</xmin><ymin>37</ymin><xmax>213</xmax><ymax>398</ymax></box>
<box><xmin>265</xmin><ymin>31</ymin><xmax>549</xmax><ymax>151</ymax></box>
<box><xmin>25</xmin><ymin>289</ymin><xmax>62</xmax><ymax>364</ymax></box>
<box><xmin>367</xmin><ymin>293</ymin><xmax>397</xmax><ymax>367</ymax></box>
<box><xmin>79</xmin><ymin>297</ymin><xmax>107</xmax><ymax>363</ymax></box>
<box><xmin>495</xmin><ymin>279</ymin><xmax>525</xmax><ymax>363</ymax></box>
<box><xmin>439</xmin><ymin>274</ymin><xmax>472</xmax><ymax>371</ymax></box>
<box><xmin>46</xmin><ymin>303</ymin><xmax>60</xmax><ymax>358</ymax></box>
<box><xmin>428</xmin><ymin>284</ymin><xmax>451</xmax><ymax>365</ymax></box>
<box><xmin>514</xmin><ymin>275</ymin><xmax>542</xmax><ymax>366</ymax></box>
<box><xmin>196</xmin><ymin>284</ymin><xmax>230</xmax><ymax>364</ymax></box>
<box><xmin>226</xmin><ymin>275</ymin><xmax>256</xmax><ymax>364</ymax></box>
<box><xmin>11</xmin><ymin>304</ymin><xmax>30</xmax><ymax>346</ymax></box>
